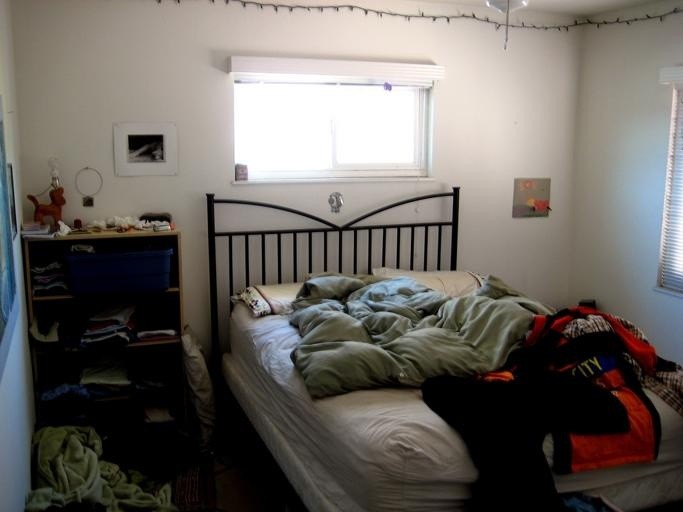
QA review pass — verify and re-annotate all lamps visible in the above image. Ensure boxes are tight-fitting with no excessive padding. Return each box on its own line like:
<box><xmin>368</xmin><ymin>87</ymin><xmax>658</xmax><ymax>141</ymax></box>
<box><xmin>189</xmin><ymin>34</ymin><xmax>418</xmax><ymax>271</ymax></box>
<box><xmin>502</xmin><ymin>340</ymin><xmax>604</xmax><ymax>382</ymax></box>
<box><xmin>26</xmin><ymin>157</ymin><xmax>66</xmax><ymax>225</ymax></box>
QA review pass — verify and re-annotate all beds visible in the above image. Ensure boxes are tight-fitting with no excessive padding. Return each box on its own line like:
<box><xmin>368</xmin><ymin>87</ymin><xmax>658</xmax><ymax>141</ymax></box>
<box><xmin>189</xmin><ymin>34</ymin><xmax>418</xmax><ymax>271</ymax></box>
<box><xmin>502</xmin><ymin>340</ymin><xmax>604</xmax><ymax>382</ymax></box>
<box><xmin>204</xmin><ymin>186</ymin><xmax>683</xmax><ymax>512</ymax></box>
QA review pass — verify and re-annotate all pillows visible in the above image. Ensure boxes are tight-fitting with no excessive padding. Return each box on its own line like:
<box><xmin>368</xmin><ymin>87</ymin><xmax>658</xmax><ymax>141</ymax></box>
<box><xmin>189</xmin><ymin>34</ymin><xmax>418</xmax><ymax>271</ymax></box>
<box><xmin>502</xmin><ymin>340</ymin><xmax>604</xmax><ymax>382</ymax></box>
<box><xmin>235</xmin><ymin>282</ymin><xmax>304</xmax><ymax>317</ymax></box>
<box><xmin>372</xmin><ymin>265</ymin><xmax>487</xmax><ymax>301</ymax></box>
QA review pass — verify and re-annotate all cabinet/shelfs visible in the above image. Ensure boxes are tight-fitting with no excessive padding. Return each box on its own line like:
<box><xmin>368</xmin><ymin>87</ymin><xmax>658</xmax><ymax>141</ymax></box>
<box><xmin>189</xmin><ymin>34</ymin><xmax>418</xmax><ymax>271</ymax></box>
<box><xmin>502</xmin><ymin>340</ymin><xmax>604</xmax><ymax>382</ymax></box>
<box><xmin>21</xmin><ymin>228</ymin><xmax>187</xmax><ymax>431</ymax></box>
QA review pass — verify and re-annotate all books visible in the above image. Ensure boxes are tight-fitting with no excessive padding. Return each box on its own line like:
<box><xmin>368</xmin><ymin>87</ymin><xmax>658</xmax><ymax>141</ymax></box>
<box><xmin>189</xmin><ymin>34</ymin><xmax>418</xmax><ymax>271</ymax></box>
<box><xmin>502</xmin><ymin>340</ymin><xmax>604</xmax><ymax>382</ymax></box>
<box><xmin>20</xmin><ymin>223</ymin><xmax>52</xmax><ymax>234</ymax></box>
<box><xmin>20</xmin><ymin>220</ymin><xmax>41</xmax><ymax>231</ymax></box>
<box><xmin>22</xmin><ymin>230</ymin><xmax>56</xmax><ymax>238</ymax></box>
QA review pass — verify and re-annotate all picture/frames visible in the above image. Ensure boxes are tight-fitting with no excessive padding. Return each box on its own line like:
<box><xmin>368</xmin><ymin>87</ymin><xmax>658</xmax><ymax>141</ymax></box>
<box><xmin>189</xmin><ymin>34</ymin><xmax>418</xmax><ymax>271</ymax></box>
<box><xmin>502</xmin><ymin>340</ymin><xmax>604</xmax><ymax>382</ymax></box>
<box><xmin>7</xmin><ymin>164</ymin><xmax>18</xmax><ymax>241</ymax></box>
<box><xmin>113</xmin><ymin>121</ymin><xmax>179</xmax><ymax>177</ymax></box>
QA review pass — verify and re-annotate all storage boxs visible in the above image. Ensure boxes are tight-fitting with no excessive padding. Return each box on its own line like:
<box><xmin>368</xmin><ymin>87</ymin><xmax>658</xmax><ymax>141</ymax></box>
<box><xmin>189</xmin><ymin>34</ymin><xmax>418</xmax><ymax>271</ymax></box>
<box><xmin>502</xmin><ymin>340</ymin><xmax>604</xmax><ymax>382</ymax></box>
<box><xmin>63</xmin><ymin>237</ymin><xmax>173</xmax><ymax>300</ymax></box>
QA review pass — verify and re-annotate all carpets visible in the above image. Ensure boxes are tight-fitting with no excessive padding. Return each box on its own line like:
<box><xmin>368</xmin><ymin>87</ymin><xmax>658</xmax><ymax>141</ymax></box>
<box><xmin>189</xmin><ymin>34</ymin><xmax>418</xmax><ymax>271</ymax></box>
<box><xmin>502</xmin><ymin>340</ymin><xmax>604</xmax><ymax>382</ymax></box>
<box><xmin>117</xmin><ymin>445</ymin><xmax>219</xmax><ymax>512</ymax></box>
